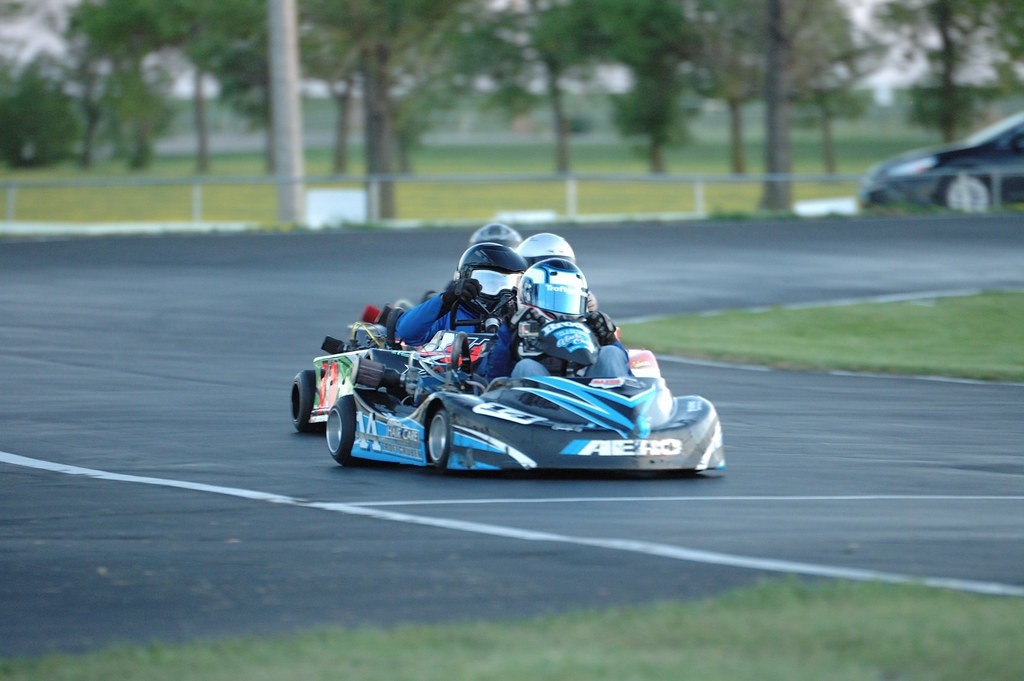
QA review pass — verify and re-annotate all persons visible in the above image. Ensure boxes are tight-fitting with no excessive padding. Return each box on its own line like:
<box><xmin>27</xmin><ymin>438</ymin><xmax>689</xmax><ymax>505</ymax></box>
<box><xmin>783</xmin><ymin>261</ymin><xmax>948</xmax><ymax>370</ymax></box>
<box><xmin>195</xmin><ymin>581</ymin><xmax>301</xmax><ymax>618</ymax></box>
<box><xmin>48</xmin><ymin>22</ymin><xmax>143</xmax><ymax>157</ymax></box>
<box><xmin>395</xmin><ymin>241</ymin><xmax>528</xmax><ymax>377</ymax></box>
<box><xmin>468</xmin><ymin>223</ymin><xmax>522</xmax><ymax>246</ymax></box>
<box><xmin>488</xmin><ymin>258</ymin><xmax>634</xmax><ymax>386</ymax></box>
<box><xmin>514</xmin><ymin>233</ymin><xmax>597</xmax><ymax>311</ymax></box>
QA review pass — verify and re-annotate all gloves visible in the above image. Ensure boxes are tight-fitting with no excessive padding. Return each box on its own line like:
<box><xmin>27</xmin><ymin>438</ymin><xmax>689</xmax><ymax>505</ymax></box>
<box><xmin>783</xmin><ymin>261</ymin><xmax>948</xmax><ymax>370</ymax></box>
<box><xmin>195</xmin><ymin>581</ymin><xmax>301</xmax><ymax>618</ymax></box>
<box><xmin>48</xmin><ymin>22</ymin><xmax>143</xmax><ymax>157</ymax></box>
<box><xmin>494</xmin><ymin>286</ymin><xmax>518</xmax><ymax>312</ymax></box>
<box><xmin>443</xmin><ymin>277</ymin><xmax>482</xmax><ymax>306</ymax></box>
<box><xmin>519</xmin><ymin>308</ymin><xmax>546</xmax><ymax>330</ymax></box>
<box><xmin>584</xmin><ymin>311</ymin><xmax>617</xmax><ymax>346</ymax></box>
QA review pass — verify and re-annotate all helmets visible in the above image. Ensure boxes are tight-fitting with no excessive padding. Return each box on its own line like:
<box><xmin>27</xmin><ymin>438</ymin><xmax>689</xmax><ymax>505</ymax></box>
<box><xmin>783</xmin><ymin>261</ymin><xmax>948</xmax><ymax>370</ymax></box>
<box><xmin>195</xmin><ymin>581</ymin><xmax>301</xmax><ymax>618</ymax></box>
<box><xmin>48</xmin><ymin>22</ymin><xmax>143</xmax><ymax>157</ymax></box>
<box><xmin>454</xmin><ymin>241</ymin><xmax>528</xmax><ymax>324</ymax></box>
<box><xmin>516</xmin><ymin>257</ymin><xmax>589</xmax><ymax>322</ymax></box>
<box><xmin>469</xmin><ymin>224</ymin><xmax>522</xmax><ymax>244</ymax></box>
<box><xmin>515</xmin><ymin>233</ymin><xmax>575</xmax><ymax>263</ymax></box>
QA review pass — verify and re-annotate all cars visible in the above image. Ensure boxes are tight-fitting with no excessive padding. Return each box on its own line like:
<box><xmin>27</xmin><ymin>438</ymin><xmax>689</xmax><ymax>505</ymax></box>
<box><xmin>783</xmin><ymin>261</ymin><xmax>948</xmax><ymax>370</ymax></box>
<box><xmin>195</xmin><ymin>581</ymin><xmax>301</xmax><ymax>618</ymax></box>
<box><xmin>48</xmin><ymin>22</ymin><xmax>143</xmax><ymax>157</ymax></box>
<box><xmin>861</xmin><ymin>111</ymin><xmax>1024</xmax><ymax>219</ymax></box>
